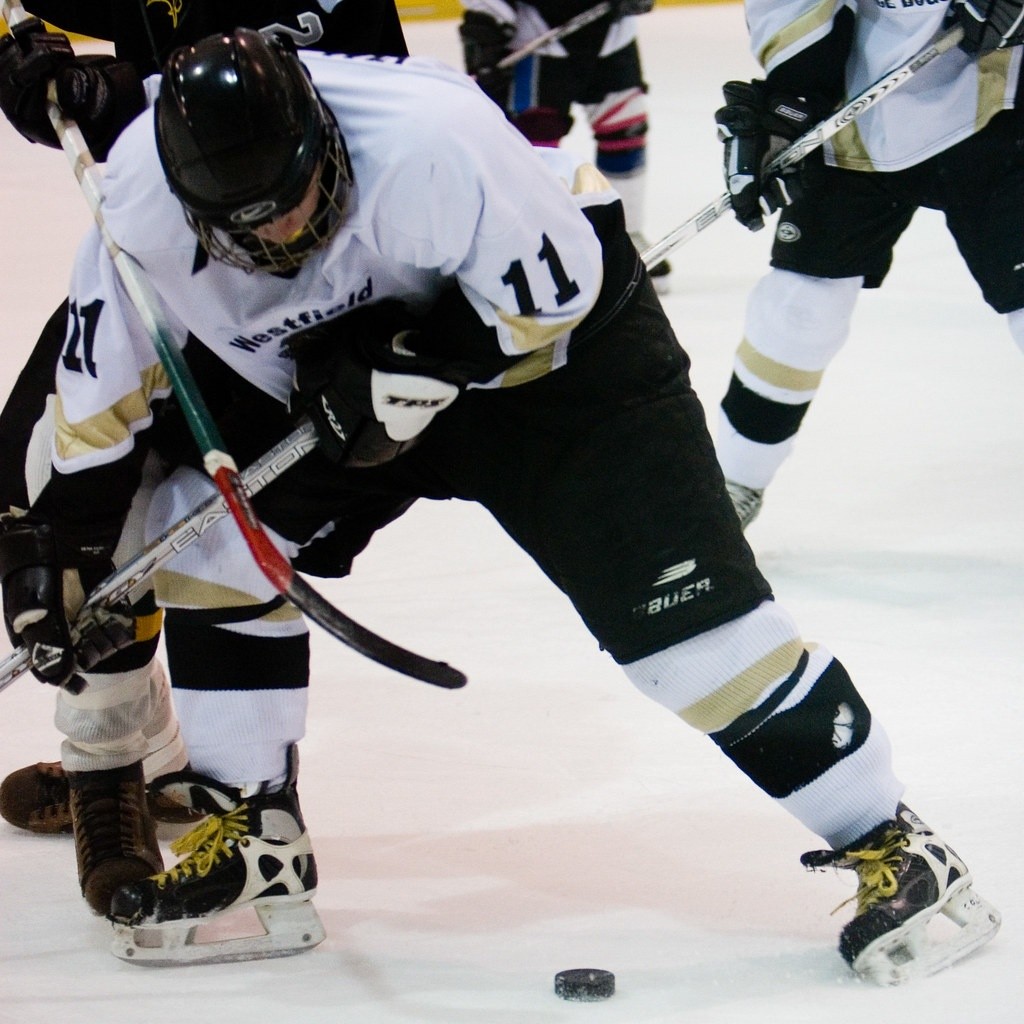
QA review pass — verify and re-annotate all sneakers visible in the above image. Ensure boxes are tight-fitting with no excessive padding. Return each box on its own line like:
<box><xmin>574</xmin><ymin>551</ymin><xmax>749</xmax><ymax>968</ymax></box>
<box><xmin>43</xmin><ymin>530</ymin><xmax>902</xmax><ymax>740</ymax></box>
<box><xmin>0</xmin><ymin>761</ymin><xmax>206</xmax><ymax>836</ymax></box>
<box><xmin>799</xmin><ymin>802</ymin><xmax>1004</xmax><ymax>986</ymax></box>
<box><xmin>69</xmin><ymin>759</ymin><xmax>165</xmax><ymax>927</ymax></box>
<box><xmin>723</xmin><ymin>477</ymin><xmax>766</xmax><ymax>530</ymax></box>
<box><xmin>112</xmin><ymin>743</ymin><xmax>325</xmax><ymax>967</ymax></box>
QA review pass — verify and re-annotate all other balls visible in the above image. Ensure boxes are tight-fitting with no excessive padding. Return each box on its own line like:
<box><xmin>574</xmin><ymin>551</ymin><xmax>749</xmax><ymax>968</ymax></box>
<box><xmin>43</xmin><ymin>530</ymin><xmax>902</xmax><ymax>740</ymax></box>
<box><xmin>554</xmin><ymin>968</ymin><xmax>616</xmax><ymax>1003</ymax></box>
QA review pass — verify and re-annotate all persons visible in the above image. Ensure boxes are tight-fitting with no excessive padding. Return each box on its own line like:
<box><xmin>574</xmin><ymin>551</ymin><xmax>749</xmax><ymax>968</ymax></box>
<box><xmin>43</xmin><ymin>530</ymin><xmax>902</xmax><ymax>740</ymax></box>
<box><xmin>708</xmin><ymin>1</ymin><xmax>1024</xmax><ymax>527</ymax></box>
<box><xmin>0</xmin><ymin>0</ymin><xmax>414</xmax><ymax>833</ymax></box>
<box><xmin>454</xmin><ymin>0</ymin><xmax>670</xmax><ymax>275</ymax></box>
<box><xmin>13</xmin><ymin>26</ymin><xmax>975</xmax><ymax>971</ymax></box>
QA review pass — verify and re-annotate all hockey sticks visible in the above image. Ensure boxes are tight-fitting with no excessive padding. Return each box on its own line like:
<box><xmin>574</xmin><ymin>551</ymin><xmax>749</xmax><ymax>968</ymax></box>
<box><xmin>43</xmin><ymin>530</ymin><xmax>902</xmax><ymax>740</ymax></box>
<box><xmin>464</xmin><ymin>2</ymin><xmax>613</xmax><ymax>81</ymax></box>
<box><xmin>0</xmin><ymin>407</ymin><xmax>318</xmax><ymax>697</ymax></box>
<box><xmin>638</xmin><ymin>22</ymin><xmax>967</xmax><ymax>273</ymax></box>
<box><xmin>35</xmin><ymin>104</ymin><xmax>470</xmax><ymax>691</ymax></box>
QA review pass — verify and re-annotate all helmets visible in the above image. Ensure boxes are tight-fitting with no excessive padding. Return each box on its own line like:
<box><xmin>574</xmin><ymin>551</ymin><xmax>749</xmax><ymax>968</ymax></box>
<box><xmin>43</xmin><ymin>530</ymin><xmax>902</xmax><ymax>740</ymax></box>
<box><xmin>154</xmin><ymin>29</ymin><xmax>353</xmax><ymax>278</ymax></box>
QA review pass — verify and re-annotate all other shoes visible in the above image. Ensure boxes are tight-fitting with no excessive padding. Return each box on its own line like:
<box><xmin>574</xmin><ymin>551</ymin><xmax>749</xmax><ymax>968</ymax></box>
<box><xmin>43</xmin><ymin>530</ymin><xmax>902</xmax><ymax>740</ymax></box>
<box><xmin>628</xmin><ymin>231</ymin><xmax>672</xmax><ymax>292</ymax></box>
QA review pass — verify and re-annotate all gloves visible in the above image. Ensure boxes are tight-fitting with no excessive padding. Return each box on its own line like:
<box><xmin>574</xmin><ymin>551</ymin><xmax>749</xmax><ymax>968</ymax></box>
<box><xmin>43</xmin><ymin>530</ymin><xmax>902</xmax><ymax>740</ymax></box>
<box><xmin>0</xmin><ymin>523</ymin><xmax>140</xmax><ymax>695</ymax></box>
<box><xmin>298</xmin><ymin>273</ymin><xmax>532</xmax><ymax>467</ymax></box>
<box><xmin>459</xmin><ymin>8</ymin><xmax>513</xmax><ymax>94</ymax></box>
<box><xmin>944</xmin><ymin>0</ymin><xmax>1024</xmax><ymax>59</ymax></box>
<box><xmin>609</xmin><ymin>0</ymin><xmax>653</xmax><ymax>22</ymax></box>
<box><xmin>715</xmin><ymin>78</ymin><xmax>830</xmax><ymax>232</ymax></box>
<box><xmin>0</xmin><ymin>17</ymin><xmax>146</xmax><ymax>163</ymax></box>
